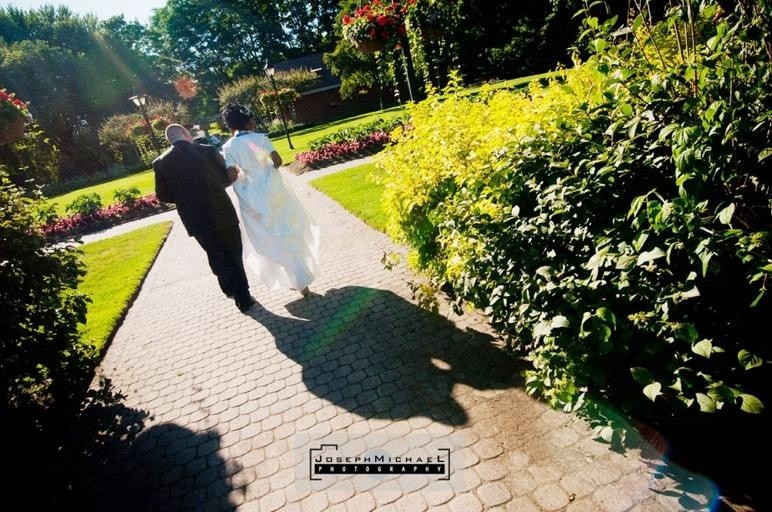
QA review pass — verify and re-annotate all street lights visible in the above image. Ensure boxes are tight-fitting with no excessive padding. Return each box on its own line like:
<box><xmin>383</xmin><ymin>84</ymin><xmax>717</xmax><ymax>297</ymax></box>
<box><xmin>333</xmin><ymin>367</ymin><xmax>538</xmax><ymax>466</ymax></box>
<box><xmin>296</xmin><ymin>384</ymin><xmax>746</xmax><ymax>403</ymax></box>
<box><xmin>263</xmin><ymin>58</ymin><xmax>294</xmax><ymax>149</ymax></box>
<box><xmin>128</xmin><ymin>86</ymin><xmax>163</xmax><ymax>156</ymax></box>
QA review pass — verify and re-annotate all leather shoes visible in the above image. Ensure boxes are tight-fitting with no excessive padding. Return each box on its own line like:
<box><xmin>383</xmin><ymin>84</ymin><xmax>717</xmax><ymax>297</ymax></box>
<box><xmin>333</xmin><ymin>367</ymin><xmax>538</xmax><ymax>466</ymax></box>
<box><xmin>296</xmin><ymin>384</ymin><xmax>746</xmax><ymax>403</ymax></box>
<box><xmin>227</xmin><ymin>290</ymin><xmax>255</xmax><ymax>312</ymax></box>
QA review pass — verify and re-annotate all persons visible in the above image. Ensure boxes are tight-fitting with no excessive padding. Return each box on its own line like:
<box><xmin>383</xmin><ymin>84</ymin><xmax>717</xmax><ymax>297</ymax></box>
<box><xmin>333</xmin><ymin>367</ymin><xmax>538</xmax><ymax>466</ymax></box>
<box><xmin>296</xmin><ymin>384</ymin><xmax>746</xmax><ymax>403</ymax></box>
<box><xmin>152</xmin><ymin>121</ymin><xmax>255</xmax><ymax>312</ymax></box>
<box><xmin>221</xmin><ymin>104</ymin><xmax>321</xmax><ymax>299</ymax></box>
